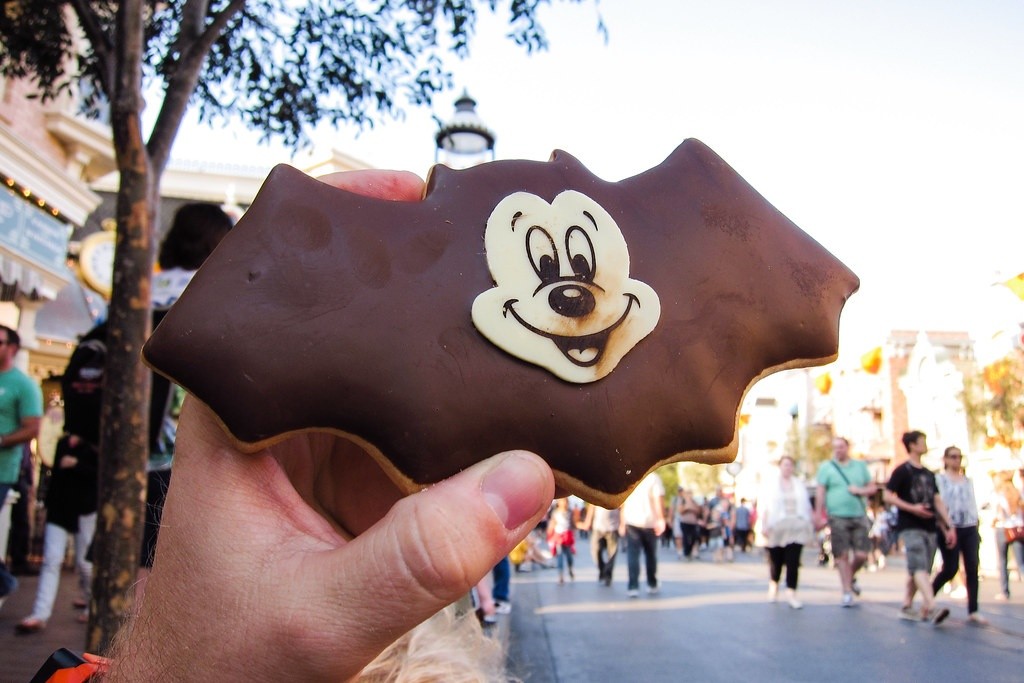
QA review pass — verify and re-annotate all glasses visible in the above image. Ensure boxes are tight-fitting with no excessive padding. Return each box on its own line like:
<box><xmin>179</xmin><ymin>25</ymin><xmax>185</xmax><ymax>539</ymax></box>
<box><xmin>947</xmin><ymin>455</ymin><xmax>962</xmax><ymax>460</ymax></box>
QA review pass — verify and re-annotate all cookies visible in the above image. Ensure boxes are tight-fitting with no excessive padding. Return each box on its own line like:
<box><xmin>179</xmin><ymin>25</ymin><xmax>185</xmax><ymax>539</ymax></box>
<box><xmin>138</xmin><ymin>137</ymin><xmax>861</xmax><ymax>511</ymax></box>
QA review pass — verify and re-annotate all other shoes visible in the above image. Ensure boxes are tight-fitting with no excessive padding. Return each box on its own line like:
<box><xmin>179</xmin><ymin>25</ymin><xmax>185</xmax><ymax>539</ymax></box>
<box><xmin>842</xmin><ymin>592</ymin><xmax>856</xmax><ymax>607</ymax></box>
<box><xmin>606</xmin><ymin>576</ymin><xmax>612</xmax><ymax>586</ymax></box>
<box><xmin>786</xmin><ymin>594</ymin><xmax>803</xmax><ymax>608</ymax></box>
<box><xmin>767</xmin><ymin>592</ymin><xmax>777</xmax><ymax>603</ymax></box>
<box><xmin>943</xmin><ymin>581</ymin><xmax>967</xmax><ymax>600</ymax></box>
<box><xmin>15</xmin><ymin>617</ymin><xmax>45</xmax><ymax>631</ymax></box>
<box><xmin>966</xmin><ymin>611</ymin><xmax>989</xmax><ymax>626</ymax></box>
<box><xmin>73</xmin><ymin>598</ymin><xmax>88</xmax><ymax>607</ymax></box>
<box><xmin>897</xmin><ymin>605</ymin><xmax>921</xmax><ymax>621</ymax></box>
<box><xmin>929</xmin><ymin>607</ymin><xmax>949</xmax><ymax>626</ymax></box>
<box><xmin>483</xmin><ymin>615</ymin><xmax>496</xmax><ymax>625</ymax></box>
<box><xmin>851</xmin><ymin>579</ymin><xmax>861</xmax><ymax>595</ymax></box>
<box><xmin>629</xmin><ymin>589</ymin><xmax>638</xmax><ymax>597</ymax></box>
<box><xmin>647</xmin><ymin>582</ymin><xmax>661</xmax><ymax>593</ymax></box>
<box><xmin>599</xmin><ymin>570</ymin><xmax>606</xmax><ymax>581</ymax></box>
<box><xmin>996</xmin><ymin>591</ymin><xmax>1011</xmax><ymax>600</ymax></box>
<box><xmin>496</xmin><ymin>602</ymin><xmax>511</xmax><ymax>614</ymax></box>
<box><xmin>79</xmin><ymin>614</ymin><xmax>89</xmax><ymax>623</ymax></box>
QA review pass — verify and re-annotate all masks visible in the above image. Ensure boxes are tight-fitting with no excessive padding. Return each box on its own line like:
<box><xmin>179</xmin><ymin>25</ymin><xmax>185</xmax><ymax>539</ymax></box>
<box><xmin>79</xmin><ymin>368</ymin><xmax>102</xmax><ymax>379</ymax></box>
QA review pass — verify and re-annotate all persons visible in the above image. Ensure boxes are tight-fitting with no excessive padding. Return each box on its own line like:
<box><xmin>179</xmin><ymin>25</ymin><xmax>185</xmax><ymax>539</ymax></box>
<box><xmin>475</xmin><ymin>556</ymin><xmax>511</xmax><ymax>622</ymax></box>
<box><xmin>754</xmin><ymin>456</ymin><xmax>814</xmax><ymax>609</ymax></box>
<box><xmin>929</xmin><ymin>445</ymin><xmax>987</xmax><ymax>624</ymax></box>
<box><xmin>988</xmin><ymin>471</ymin><xmax>1024</xmax><ymax>599</ymax></box>
<box><xmin>29</xmin><ymin>168</ymin><xmax>556</xmax><ymax>683</ymax></box>
<box><xmin>881</xmin><ymin>430</ymin><xmax>957</xmax><ymax>623</ymax></box>
<box><xmin>815</xmin><ymin>437</ymin><xmax>887</xmax><ymax>606</ymax></box>
<box><xmin>17</xmin><ymin>433</ymin><xmax>101</xmax><ymax>629</ymax></box>
<box><xmin>865</xmin><ymin>502</ymin><xmax>890</xmax><ymax>570</ymax></box>
<box><xmin>0</xmin><ymin>323</ymin><xmax>44</xmax><ymax>604</ymax></box>
<box><xmin>548</xmin><ymin>497</ymin><xmax>621</xmax><ymax>585</ymax></box>
<box><xmin>618</xmin><ymin>472</ymin><xmax>665</xmax><ymax>596</ymax></box>
<box><xmin>670</xmin><ymin>485</ymin><xmax>751</xmax><ymax>562</ymax></box>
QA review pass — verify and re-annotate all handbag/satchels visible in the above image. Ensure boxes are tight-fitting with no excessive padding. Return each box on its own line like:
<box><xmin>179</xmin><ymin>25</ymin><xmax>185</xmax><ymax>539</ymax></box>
<box><xmin>1004</xmin><ymin>516</ymin><xmax>1024</xmax><ymax>543</ymax></box>
<box><xmin>868</xmin><ymin>511</ymin><xmax>897</xmax><ymax>556</ymax></box>
